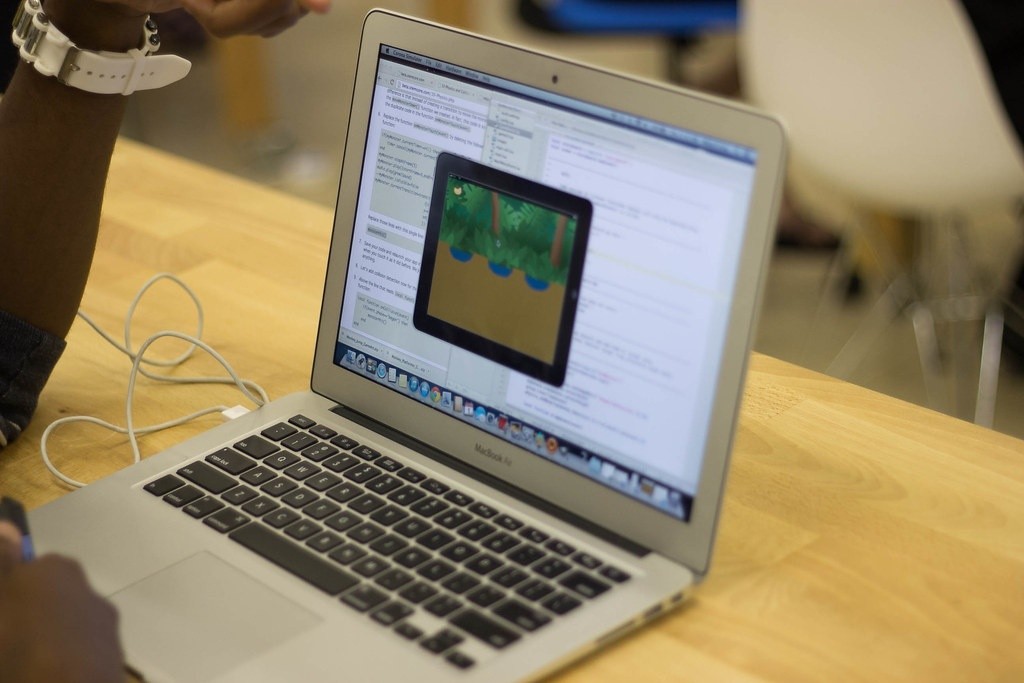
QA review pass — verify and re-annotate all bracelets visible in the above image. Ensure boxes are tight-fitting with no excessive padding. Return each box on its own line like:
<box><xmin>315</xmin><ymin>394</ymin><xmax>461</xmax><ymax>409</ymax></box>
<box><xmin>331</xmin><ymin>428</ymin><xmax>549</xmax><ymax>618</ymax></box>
<box><xmin>10</xmin><ymin>0</ymin><xmax>192</xmax><ymax>96</ymax></box>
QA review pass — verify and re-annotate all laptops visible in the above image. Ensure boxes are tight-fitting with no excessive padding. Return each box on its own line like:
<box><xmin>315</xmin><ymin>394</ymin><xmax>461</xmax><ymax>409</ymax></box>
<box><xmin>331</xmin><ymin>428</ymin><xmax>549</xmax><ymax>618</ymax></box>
<box><xmin>31</xmin><ymin>6</ymin><xmax>786</xmax><ymax>682</ymax></box>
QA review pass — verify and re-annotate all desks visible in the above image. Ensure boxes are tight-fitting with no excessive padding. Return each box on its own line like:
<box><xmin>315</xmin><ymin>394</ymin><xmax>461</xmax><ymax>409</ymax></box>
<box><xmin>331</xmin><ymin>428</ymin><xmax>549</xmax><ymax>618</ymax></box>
<box><xmin>0</xmin><ymin>133</ymin><xmax>1024</xmax><ymax>683</ymax></box>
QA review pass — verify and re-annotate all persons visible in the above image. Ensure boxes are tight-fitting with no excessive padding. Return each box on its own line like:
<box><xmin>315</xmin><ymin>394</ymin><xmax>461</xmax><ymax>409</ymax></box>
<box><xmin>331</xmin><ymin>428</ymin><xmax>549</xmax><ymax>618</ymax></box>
<box><xmin>0</xmin><ymin>0</ymin><xmax>334</xmax><ymax>451</ymax></box>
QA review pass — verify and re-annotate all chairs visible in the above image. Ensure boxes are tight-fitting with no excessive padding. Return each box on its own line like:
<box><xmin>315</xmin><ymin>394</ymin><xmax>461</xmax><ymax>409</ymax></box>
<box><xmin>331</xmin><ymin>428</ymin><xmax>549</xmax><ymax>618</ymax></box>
<box><xmin>730</xmin><ymin>0</ymin><xmax>1024</xmax><ymax>433</ymax></box>
